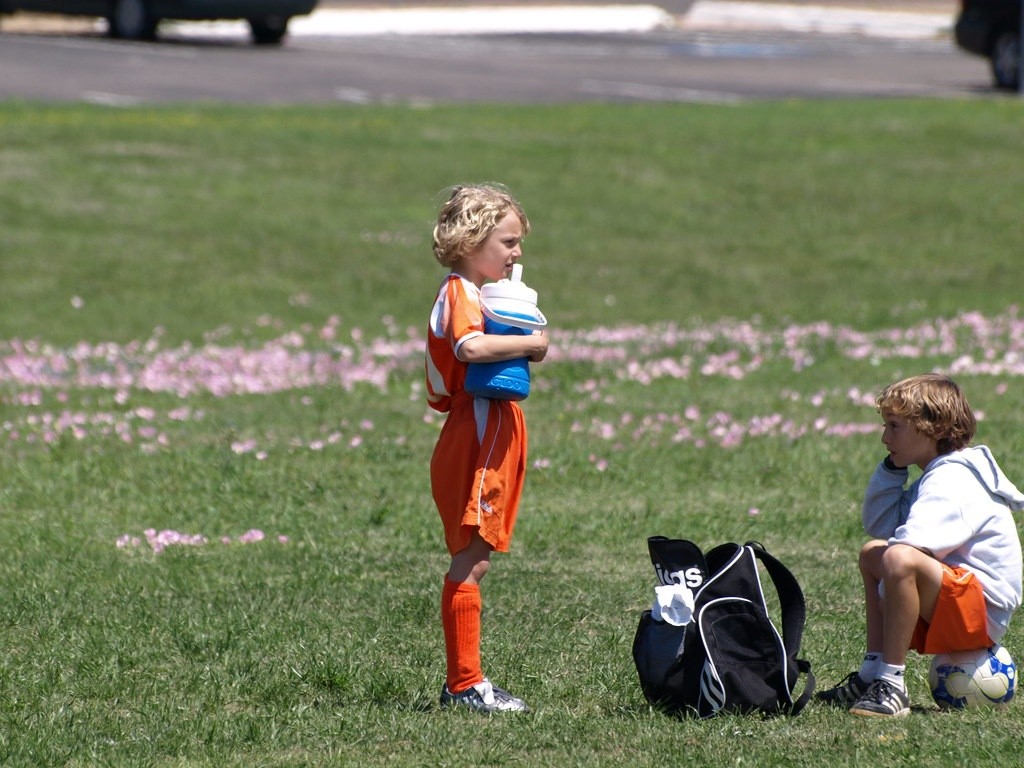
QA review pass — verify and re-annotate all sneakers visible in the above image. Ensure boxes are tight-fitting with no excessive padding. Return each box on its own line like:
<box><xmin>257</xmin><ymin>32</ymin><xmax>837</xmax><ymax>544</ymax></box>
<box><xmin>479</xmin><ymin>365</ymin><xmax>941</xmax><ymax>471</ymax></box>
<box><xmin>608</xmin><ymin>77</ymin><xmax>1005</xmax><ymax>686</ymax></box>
<box><xmin>848</xmin><ymin>679</ymin><xmax>911</xmax><ymax>721</ymax></box>
<box><xmin>440</xmin><ymin>677</ymin><xmax>527</xmax><ymax>714</ymax></box>
<box><xmin>815</xmin><ymin>672</ymin><xmax>872</xmax><ymax>707</ymax></box>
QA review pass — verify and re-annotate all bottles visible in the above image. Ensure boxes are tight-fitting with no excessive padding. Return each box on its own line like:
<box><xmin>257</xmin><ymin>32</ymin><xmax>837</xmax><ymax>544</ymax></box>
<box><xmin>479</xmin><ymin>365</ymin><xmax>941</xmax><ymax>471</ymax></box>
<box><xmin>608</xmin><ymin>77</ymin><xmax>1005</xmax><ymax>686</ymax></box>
<box><xmin>464</xmin><ymin>263</ymin><xmax>539</xmax><ymax>402</ymax></box>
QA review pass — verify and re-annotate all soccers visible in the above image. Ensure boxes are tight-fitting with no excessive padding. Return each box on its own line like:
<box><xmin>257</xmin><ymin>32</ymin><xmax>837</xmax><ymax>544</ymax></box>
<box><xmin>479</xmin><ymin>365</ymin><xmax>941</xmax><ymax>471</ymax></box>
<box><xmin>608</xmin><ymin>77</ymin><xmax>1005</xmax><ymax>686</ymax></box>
<box><xmin>927</xmin><ymin>642</ymin><xmax>1019</xmax><ymax>710</ymax></box>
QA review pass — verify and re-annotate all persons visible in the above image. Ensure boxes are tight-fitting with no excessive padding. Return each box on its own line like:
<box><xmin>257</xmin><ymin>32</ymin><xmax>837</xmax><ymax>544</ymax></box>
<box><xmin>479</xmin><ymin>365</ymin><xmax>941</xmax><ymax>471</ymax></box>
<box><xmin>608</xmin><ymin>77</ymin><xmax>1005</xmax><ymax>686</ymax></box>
<box><xmin>814</xmin><ymin>372</ymin><xmax>1023</xmax><ymax>717</ymax></box>
<box><xmin>423</xmin><ymin>181</ymin><xmax>551</xmax><ymax>713</ymax></box>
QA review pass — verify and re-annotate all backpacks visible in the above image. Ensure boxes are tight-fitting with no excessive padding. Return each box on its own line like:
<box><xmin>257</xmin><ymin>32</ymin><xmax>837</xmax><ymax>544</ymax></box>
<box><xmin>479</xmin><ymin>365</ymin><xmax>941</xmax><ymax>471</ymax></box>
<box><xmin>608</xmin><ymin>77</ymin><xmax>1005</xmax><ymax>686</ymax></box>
<box><xmin>631</xmin><ymin>536</ymin><xmax>815</xmax><ymax>720</ymax></box>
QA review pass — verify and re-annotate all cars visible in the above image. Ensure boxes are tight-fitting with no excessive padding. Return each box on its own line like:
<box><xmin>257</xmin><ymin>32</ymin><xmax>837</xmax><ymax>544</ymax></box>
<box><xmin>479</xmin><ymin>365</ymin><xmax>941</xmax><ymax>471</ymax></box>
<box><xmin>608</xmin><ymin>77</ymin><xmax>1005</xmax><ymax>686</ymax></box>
<box><xmin>0</xmin><ymin>0</ymin><xmax>322</xmax><ymax>43</ymax></box>
<box><xmin>950</xmin><ymin>0</ymin><xmax>1024</xmax><ymax>92</ymax></box>
